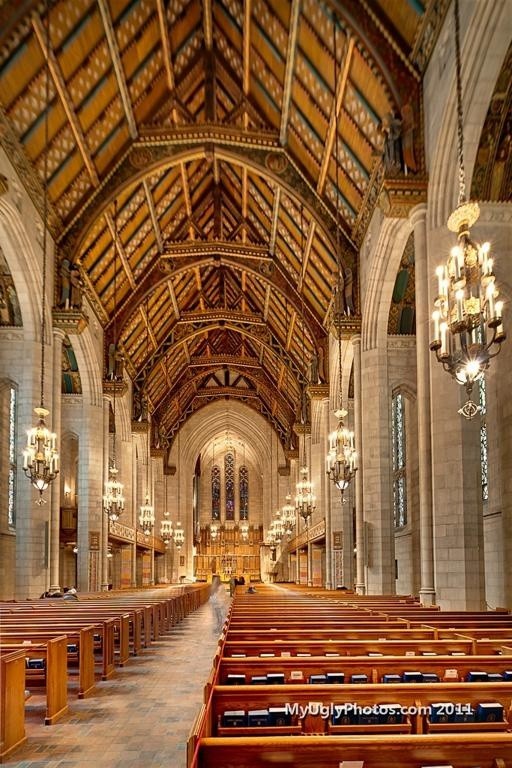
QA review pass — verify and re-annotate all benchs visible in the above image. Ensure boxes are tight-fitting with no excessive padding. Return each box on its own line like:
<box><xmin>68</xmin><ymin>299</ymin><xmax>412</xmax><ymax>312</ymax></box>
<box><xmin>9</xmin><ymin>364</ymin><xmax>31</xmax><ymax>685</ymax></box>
<box><xmin>187</xmin><ymin>583</ymin><xmax>512</xmax><ymax>768</ymax></box>
<box><xmin>1</xmin><ymin>584</ymin><xmax>212</xmax><ymax>762</ymax></box>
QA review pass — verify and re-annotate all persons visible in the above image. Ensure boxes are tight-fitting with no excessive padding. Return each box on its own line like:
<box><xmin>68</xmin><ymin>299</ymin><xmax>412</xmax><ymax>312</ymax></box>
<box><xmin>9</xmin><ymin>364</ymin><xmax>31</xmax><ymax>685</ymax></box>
<box><xmin>208</xmin><ymin>575</ymin><xmax>228</xmax><ymax>634</ymax></box>
<box><xmin>230</xmin><ymin>575</ymin><xmax>245</xmax><ymax>597</ymax></box>
<box><xmin>246</xmin><ymin>586</ymin><xmax>258</xmax><ymax>593</ymax></box>
<box><xmin>252</xmin><ymin>586</ymin><xmax>255</xmax><ymax>589</ymax></box>
<box><xmin>63</xmin><ymin>588</ymin><xmax>79</xmax><ymax>599</ymax></box>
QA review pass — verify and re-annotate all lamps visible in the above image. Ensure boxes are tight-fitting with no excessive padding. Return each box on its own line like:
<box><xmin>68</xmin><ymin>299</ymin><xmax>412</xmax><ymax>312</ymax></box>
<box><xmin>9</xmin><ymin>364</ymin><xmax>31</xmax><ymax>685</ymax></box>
<box><xmin>260</xmin><ymin>0</ymin><xmax>366</xmax><ymax>553</ymax></box>
<box><xmin>428</xmin><ymin>1</ymin><xmax>509</xmax><ymax>421</ymax></box>
<box><xmin>20</xmin><ymin>112</ymin><xmax>186</xmax><ymax>553</ymax></box>
